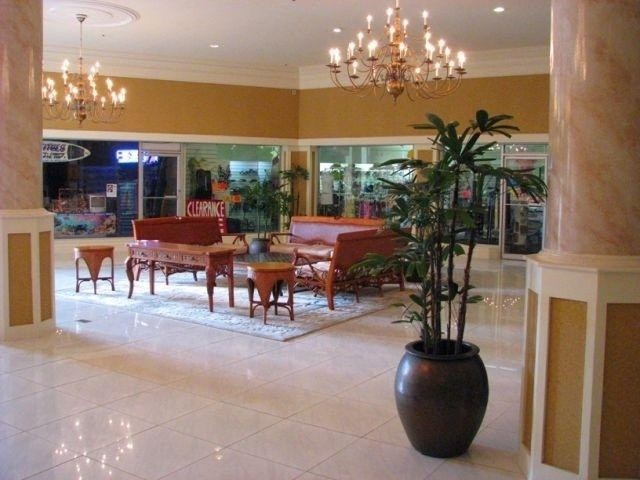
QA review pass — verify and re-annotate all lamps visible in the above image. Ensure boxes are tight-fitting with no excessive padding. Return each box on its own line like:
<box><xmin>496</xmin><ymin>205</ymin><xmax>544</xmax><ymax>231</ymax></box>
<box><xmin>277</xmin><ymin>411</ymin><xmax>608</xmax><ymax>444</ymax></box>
<box><xmin>327</xmin><ymin>0</ymin><xmax>468</xmax><ymax>105</ymax></box>
<box><xmin>42</xmin><ymin>0</ymin><xmax>141</xmax><ymax>124</ymax></box>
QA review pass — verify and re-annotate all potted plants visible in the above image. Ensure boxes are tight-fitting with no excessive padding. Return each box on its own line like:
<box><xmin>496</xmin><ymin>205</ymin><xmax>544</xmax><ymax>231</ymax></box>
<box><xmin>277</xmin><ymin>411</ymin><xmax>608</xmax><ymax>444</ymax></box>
<box><xmin>342</xmin><ymin>111</ymin><xmax>548</xmax><ymax>458</ymax></box>
<box><xmin>239</xmin><ymin>164</ymin><xmax>309</xmax><ymax>253</ymax></box>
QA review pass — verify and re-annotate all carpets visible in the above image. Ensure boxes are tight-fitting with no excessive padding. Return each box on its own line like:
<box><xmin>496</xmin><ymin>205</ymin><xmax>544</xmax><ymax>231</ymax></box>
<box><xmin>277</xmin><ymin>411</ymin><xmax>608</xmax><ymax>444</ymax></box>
<box><xmin>55</xmin><ymin>270</ymin><xmax>463</xmax><ymax>341</ymax></box>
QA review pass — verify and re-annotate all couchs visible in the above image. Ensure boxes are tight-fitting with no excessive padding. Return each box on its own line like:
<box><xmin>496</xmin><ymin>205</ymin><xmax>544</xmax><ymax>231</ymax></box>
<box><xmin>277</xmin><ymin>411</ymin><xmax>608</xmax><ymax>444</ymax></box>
<box><xmin>131</xmin><ymin>215</ymin><xmax>249</xmax><ymax>285</ymax></box>
<box><xmin>267</xmin><ymin>216</ymin><xmax>385</xmax><ymax>260</ymax></box>
<box><xmin>292</xmin><ymin>228</ymin><xmax>411</xmax><ymax>310</ymax></box>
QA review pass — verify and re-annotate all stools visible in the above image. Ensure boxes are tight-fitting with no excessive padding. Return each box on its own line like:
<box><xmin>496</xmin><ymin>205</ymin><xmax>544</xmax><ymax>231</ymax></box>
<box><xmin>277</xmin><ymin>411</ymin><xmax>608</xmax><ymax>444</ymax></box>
<box><xmin>75</xmin><ymin>245</ymin><xmax>114</xmax><ymax>295</ymax></box>
<box><xmin>247</xmin><ymin>262</ymin><xmax>295</xmax><ymax>324</ymax></box>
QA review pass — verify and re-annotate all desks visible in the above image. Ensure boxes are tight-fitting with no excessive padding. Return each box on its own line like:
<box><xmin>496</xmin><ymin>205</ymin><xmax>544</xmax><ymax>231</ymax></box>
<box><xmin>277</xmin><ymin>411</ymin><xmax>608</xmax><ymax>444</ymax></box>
<box><xmin>126</xmin><ymin>240</ymin><xmax>236</xmax><ymax>312</ymax></box>
